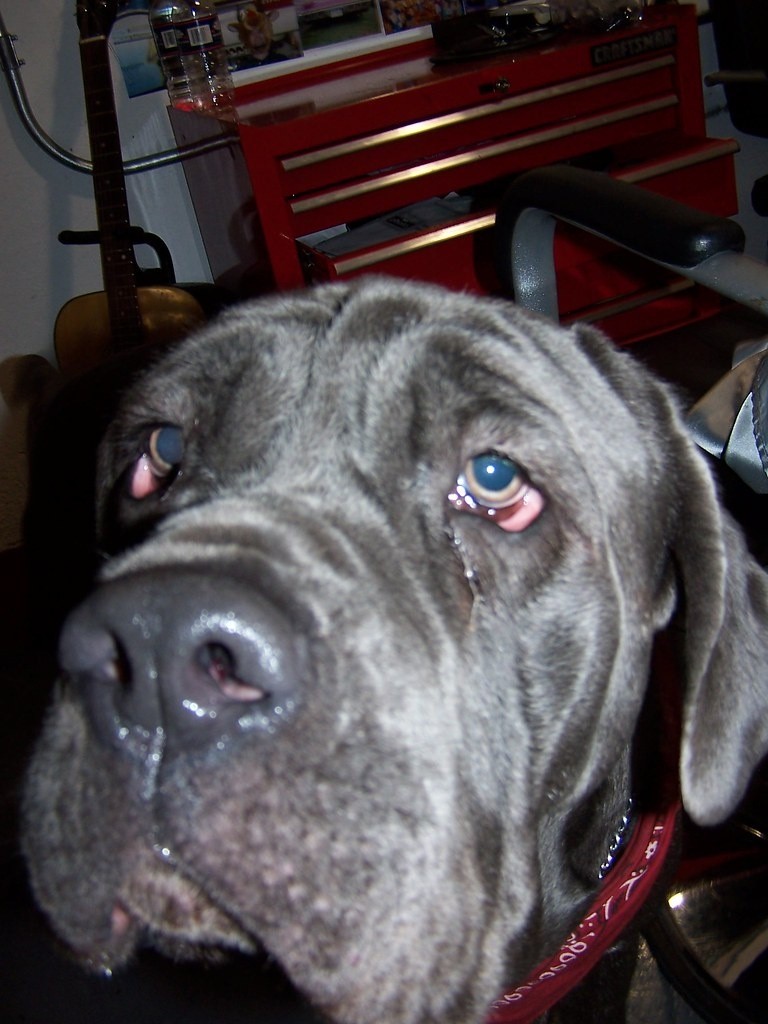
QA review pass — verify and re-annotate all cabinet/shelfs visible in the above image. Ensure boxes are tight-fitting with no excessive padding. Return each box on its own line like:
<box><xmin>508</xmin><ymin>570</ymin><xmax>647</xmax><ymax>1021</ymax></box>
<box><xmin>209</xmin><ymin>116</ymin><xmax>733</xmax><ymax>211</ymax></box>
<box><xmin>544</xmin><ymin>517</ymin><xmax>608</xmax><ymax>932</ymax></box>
<box><xmin>166</xmin><ymin>4</ymin><xmax>741</xmax><ymax>349</ymax></box>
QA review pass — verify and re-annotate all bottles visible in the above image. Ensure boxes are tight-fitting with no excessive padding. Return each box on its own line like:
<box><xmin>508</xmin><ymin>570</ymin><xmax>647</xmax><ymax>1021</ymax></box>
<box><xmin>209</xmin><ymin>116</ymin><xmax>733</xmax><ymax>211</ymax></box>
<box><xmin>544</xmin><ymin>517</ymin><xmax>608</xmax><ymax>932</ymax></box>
<box><xmin>171</xmin><ymin>0</ymin><xmax>238</xmax><ymax>123</ymax></box>
<box><xmin>148</xmin><ymin>0</ymin><xmax>194</xmax><ymax>109</ymax></box>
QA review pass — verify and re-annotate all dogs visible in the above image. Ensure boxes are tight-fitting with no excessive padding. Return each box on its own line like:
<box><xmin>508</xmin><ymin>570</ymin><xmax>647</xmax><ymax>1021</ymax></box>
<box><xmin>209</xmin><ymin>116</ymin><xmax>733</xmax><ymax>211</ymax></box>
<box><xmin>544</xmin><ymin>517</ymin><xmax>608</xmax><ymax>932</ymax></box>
<box><xmin>20</xmin><ymin>271</ymin><xmax>767</xmax><ymax>1023</ymax></box>
